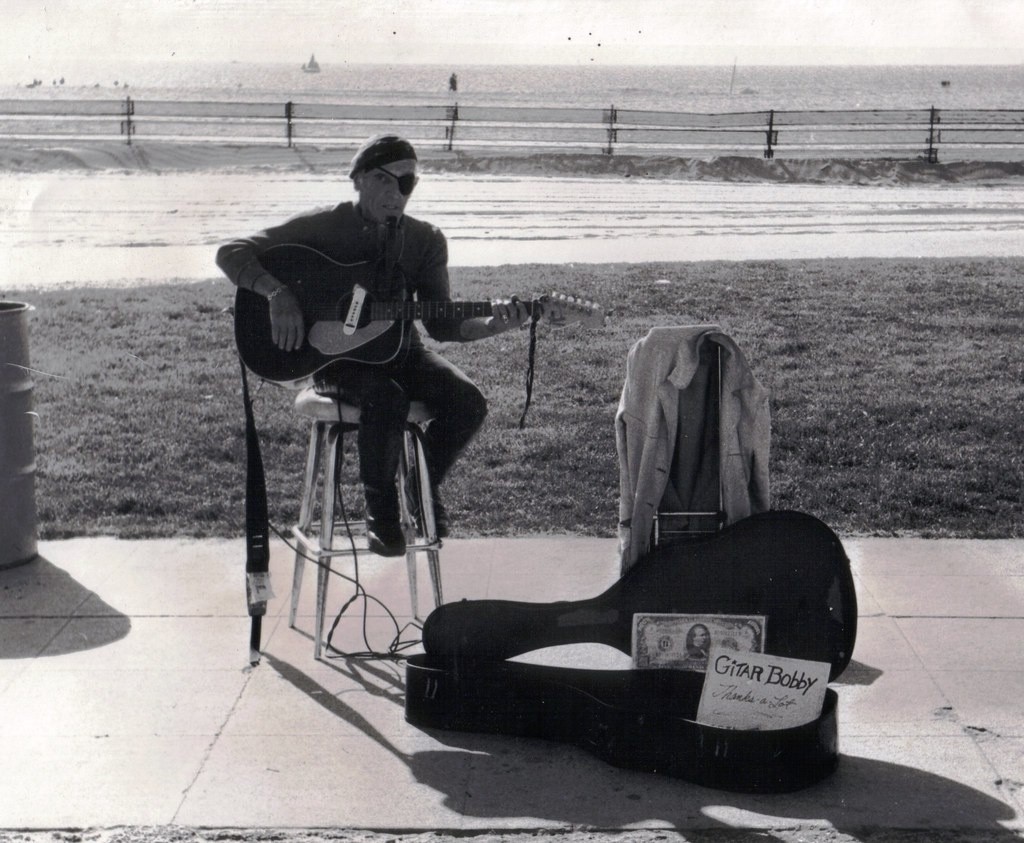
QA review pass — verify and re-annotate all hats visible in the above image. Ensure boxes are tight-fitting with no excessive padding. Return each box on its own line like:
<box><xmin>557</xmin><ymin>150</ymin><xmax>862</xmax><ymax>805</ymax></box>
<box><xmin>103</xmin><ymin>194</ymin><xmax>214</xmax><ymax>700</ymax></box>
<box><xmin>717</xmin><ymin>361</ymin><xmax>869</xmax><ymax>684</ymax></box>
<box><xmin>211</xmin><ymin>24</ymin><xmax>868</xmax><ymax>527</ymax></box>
<box><xmin>349</xmin><ymin>133</ymin><xmax>418</xmax><ymax>179</ymax></box>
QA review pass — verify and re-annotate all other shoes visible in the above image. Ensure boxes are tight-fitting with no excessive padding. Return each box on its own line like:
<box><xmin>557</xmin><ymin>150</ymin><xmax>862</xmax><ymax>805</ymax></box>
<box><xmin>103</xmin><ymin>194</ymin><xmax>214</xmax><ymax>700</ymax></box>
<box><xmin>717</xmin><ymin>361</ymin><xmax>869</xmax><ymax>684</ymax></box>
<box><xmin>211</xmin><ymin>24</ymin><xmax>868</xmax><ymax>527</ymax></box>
<box><xmin>363</xmin><ymin>499</ymin><xmax>406</xmax><ymax>556</ymax></box>
<box><xmin>404</xmin><ymin>484</ymin><xmax>449</xmax><ymax>537</ymax></box>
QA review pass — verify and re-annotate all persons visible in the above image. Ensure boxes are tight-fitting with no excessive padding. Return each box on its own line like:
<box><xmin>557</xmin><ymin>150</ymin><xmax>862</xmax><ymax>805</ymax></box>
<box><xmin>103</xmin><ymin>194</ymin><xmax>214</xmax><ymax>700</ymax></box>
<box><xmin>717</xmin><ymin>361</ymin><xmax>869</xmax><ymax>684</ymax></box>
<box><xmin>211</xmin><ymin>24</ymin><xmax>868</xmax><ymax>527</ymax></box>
<box><xmin>449</xmin><ymin>73</ymin><xmax>457</xmax><ymax>92</ymax></box>
<box><xmin>686</xmin><ymin>624</ymin><xmax>711</xmax><ymax>659</ymax></box>
<box><xmin>214</xmin><ymin>132</ymin><xmax>529</xmax><ymax>558</ymax></box>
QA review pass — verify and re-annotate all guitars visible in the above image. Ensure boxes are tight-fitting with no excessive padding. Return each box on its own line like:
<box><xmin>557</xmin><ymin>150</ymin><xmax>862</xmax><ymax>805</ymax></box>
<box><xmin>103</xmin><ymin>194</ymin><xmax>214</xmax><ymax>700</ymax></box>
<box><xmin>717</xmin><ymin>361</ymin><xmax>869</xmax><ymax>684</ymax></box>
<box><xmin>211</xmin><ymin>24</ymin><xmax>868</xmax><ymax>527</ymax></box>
<box><xmin>233</xmin><ymin>242</ymin><xmax>608</xmax><ymax>386</ymax></box>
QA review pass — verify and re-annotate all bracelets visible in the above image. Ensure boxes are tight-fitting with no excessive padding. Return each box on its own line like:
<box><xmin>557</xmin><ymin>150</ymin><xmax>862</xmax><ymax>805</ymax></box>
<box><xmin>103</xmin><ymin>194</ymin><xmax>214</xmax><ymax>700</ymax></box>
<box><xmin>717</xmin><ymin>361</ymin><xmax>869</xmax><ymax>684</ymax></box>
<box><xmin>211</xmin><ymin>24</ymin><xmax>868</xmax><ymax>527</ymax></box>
<box><xmin>266</xmin><ymin>284</ymin><xmax>288</xmax><ymax>301</ymax></box>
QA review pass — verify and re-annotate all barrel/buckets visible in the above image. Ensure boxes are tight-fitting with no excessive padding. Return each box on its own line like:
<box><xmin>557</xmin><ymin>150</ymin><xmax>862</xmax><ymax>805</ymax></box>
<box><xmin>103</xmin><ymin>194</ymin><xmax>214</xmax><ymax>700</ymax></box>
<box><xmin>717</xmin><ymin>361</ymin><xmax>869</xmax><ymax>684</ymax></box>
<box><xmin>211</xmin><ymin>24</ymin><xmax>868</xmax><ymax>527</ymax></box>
<box><xmin>0</xmin><ymin>301</ymin><xmax>39</xmax><ymax>570</ymax></box>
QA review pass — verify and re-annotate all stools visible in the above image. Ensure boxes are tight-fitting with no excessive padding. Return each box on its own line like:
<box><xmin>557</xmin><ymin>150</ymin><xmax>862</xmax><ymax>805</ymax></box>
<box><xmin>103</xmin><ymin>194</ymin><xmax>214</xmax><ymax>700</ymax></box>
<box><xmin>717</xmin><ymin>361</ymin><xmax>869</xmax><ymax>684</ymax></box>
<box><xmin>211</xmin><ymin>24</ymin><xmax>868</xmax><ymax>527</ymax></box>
<box><xmin>288</xmin><ymin>387</ymin><xmax>445</xmax><ymax>662</ymax></box>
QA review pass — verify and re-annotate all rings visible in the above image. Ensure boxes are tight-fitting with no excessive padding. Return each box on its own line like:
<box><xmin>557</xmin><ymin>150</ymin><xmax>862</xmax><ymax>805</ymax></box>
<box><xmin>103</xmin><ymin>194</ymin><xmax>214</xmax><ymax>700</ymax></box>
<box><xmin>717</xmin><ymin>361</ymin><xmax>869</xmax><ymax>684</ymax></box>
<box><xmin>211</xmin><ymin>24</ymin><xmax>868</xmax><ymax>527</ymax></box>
<box><xmin>502</xmin><ymin>314</ymin><xmax>508</xmax><ymax>324</ymax></box>
